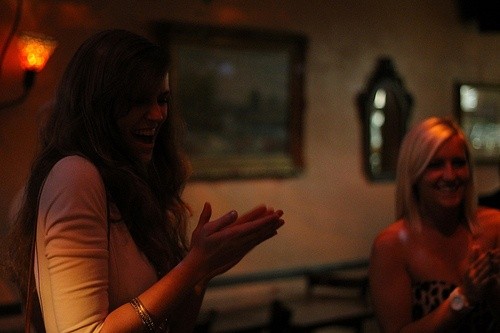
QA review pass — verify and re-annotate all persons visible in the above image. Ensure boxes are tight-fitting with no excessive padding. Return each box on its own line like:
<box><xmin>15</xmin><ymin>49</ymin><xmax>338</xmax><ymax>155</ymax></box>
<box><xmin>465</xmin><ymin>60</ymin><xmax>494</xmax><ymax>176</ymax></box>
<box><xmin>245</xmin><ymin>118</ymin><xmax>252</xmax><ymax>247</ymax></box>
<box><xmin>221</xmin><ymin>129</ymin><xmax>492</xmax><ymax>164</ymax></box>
<box><xmin>5</xmin><ymin>28</ymin><xmax>285</xmax><ymax>333</ymax></box>
<box><xmin>367</xmin><ymin>115</ymin><xmax>500</xmax><ymax>333</ymax></box>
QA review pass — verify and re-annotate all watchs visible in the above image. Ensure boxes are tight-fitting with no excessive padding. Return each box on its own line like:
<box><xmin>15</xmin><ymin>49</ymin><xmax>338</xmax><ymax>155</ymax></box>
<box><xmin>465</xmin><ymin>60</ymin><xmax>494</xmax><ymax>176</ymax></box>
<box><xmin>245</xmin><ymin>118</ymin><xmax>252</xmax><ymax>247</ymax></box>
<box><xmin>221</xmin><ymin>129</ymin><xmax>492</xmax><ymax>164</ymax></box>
<box><xmin>450</xmin><ymin>293</ymin><xmax>470</xmax><ymax>312</ymax></box>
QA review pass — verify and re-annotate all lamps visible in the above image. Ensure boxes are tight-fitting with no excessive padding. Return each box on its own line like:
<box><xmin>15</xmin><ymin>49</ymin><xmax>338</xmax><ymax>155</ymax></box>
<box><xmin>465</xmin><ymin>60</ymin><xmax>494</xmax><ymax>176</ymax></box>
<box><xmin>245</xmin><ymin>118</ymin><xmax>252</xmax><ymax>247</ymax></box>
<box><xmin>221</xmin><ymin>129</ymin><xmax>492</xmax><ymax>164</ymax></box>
<box><xmin>0</xmin><ymin>30</ymin><xmax>62</xmax><ymax>109</ymax></box>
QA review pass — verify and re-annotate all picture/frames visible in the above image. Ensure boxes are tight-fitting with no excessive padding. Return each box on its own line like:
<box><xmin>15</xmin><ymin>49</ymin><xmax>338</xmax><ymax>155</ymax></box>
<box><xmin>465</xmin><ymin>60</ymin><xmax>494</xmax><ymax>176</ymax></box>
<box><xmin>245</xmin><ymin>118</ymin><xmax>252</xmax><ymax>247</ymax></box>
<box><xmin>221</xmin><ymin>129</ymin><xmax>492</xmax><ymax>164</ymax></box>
<box><xmin>148</xmin><ymin>17</ymin><xmax>308</xmax><ymax>181</ymax></box>
<box><xmin>453</xmin><ymin>81</ymin><xmax>500</xmax><ymax>196</ymax></box>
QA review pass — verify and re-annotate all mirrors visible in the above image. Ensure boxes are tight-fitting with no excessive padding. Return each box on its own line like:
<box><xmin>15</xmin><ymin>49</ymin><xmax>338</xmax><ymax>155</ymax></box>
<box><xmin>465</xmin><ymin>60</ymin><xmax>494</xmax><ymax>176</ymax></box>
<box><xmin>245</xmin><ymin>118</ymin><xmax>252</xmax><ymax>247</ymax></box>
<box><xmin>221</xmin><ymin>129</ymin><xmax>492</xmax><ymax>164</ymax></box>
<box><xmin>356</xmin><ymin>57</ymin><xmax>415</xmax><ymax>181</ymax></box>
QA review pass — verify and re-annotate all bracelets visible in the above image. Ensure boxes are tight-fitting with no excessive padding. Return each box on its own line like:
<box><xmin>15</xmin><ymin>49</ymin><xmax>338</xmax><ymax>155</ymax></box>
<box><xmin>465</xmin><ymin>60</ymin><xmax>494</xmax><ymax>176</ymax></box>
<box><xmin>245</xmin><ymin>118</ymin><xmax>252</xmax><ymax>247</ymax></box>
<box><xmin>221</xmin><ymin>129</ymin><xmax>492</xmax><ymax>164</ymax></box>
<box><xmin>129</xmin><ymin>296</ymin><xmax>166</xmax><ymax>333</ymax></box>
